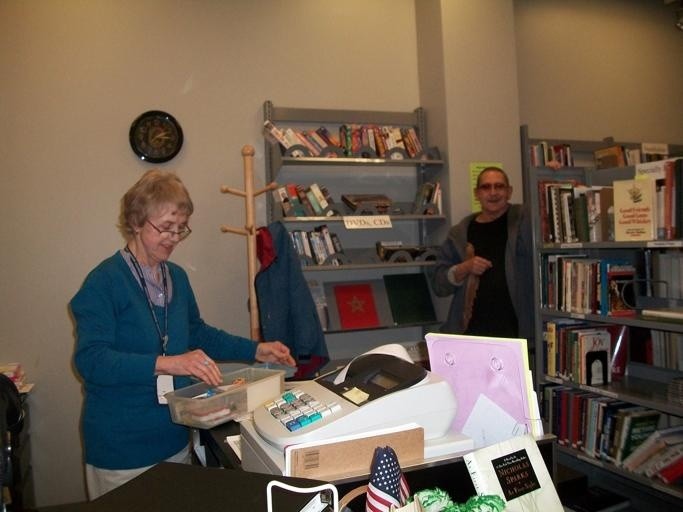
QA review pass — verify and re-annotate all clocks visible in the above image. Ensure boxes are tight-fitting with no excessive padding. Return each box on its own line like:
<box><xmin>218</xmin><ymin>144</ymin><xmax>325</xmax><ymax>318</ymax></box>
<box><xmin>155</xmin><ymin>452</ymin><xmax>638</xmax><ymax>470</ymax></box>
<box><xmin>129</xmin><ymin>110</ymin><xmax>183</xmax><ymax>164</ymax></box>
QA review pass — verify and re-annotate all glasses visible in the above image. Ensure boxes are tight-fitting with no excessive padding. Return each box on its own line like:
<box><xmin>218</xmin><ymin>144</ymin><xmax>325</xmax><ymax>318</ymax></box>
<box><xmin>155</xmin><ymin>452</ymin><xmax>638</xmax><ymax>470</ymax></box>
<box><xmin>148</xmin><ymin>220</ymin><xmax>192</xmax><ymax>241</ymax></box>
<box><xmin>478</xmin><ymin>182</ymin><xmax>509</xmax><ymax>191</ymax></box>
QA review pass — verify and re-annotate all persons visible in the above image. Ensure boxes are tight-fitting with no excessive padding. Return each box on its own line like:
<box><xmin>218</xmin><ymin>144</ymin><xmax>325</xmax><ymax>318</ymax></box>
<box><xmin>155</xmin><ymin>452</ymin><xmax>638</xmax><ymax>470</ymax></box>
<box><xmin>424</xmin><ymin>167</ymin><xmax>534</xmax><ymax>351</ymax></box>
<box><xmin>69</xmin><ymin>168</ymin><xmax>298</xmax><ymax>506</ymax></box>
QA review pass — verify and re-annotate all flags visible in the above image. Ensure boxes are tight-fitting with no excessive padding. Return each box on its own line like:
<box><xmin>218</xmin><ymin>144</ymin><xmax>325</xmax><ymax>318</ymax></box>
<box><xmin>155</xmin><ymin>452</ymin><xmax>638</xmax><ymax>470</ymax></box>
<box><xmin>361</xmin><ymin>446</ymin><xmax>413</xmax><ymax>510</ymax></box>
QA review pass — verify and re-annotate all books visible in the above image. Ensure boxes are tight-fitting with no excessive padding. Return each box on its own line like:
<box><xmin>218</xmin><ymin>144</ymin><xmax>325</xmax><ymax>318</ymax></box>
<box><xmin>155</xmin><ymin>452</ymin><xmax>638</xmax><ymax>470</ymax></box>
<box><xmin>0</xmin><ymin>360</ymin><xmax>36</xmax><ymax>394</ymax></box>
<box><xmin>460</xmin><ymin>430</ymin><xmax>566</xmax><ymax>512</ymax></box>
<box><xmin>538</xmin><ymin>252</ymin><xmax>683</xmax><ymax>320</ymax></box>
<box><xmin>263</xmin><ymin>120</ymin><xmax>425</xmax><ymax>160</ymax></box>
<box><xmin>533</xmin><ymin>139</ymin><xmax>682</xmax><ymax>243</ymax></box>
<box><xmin>544</xmin><ymin>317</ymin><xmax>680</xmax><ymax>386</ymax></box>
<box><xmin>308</xmin><ymin>274</ymin><xmax>437</xmax><ymax>329</ymax></box>
<box><xmin>421</xmin><ymin>332</ymin><xmax>543</xmax><ymax>439</ymax></box>
<box><xmin>290</xmin><ymin>225</ymin><xmax>402</xmax><ymax>267</ymax></box>
<box><xmin>539</xmin><ymin>381</ymin><xmax>682</xmax><ymax>483</ymax></box>
<box><xmin>272</xmin><ymin>183</ymin><xmax>443</xmax><ymax>217</ymax></box>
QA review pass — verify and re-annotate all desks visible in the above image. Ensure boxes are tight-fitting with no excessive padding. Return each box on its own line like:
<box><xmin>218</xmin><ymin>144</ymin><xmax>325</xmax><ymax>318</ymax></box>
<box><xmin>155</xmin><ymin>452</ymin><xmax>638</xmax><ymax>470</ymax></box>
<box><xmin>204</xmin><ymin>420</ymin><xmax>556</xmax><ymax>502</ymax></box>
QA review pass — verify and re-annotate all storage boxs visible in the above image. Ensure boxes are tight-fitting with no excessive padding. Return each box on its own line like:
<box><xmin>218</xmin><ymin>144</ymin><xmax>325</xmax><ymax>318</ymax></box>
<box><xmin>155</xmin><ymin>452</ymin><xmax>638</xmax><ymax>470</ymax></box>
<box><xmin>164</xmin><ymin>367</ymin><xmax>287</xmax><ymax>429</ymax></box>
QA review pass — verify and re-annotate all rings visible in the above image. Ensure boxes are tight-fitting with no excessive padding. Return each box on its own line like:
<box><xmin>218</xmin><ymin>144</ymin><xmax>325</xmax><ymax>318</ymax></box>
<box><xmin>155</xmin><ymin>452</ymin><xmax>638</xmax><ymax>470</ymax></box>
<box><xmin>202</xmin><ymin>360</ymin><xmax>210</xmax><ymax>367</ymax></box>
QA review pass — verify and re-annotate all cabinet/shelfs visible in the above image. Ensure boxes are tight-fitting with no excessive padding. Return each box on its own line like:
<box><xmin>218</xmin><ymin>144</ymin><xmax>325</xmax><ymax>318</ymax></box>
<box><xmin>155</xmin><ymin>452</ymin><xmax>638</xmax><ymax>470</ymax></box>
<box><xmin>520</xmin><ymin>124</ymin><xmax>683</xmax><ymax>512</ymax></box>
<box><xmin>263</xmin><ymin>99</ymin><xmax>447</xmax><ymax>362</ymax></box>
